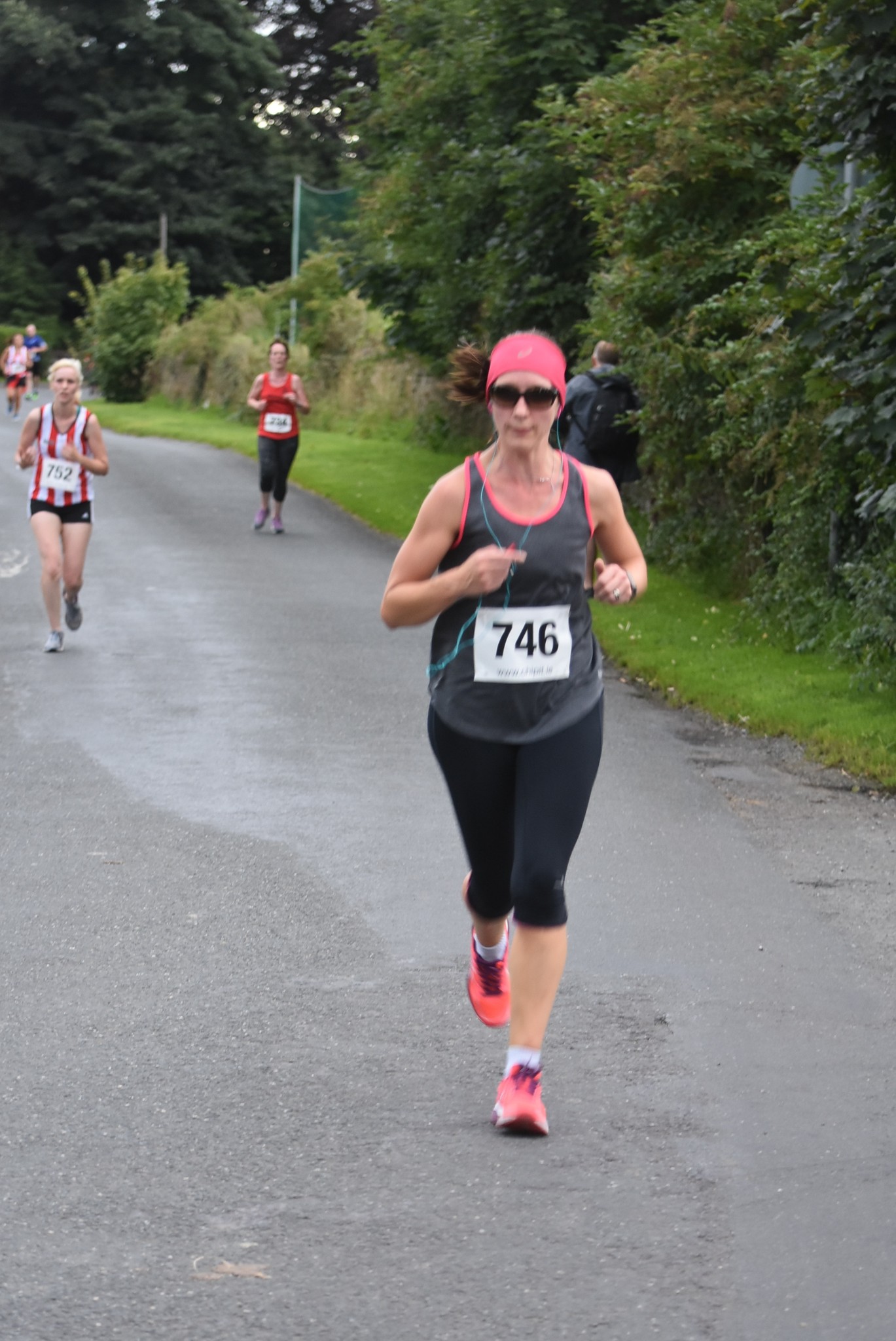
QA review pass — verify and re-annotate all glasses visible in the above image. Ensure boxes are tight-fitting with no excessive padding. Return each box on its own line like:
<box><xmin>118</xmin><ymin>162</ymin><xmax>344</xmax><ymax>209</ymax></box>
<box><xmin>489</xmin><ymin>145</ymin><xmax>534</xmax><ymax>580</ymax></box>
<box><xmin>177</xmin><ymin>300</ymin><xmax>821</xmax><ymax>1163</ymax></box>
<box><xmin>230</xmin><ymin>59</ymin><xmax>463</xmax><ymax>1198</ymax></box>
<box><xmin>490</xmin><ymin>386</ymin><xmax>558</xmax><ymax>412</ymax></box>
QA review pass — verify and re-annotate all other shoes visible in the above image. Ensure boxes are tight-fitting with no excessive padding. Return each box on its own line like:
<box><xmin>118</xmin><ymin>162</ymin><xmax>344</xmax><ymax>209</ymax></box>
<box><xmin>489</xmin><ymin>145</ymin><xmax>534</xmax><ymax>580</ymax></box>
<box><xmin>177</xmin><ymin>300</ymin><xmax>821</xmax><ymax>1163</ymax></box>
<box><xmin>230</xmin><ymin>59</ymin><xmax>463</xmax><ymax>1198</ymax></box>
<box><xmin>43</xmin><ymin>631</ymin><xmax>65</xmax><ymax>652</ymax></box>
<box><xmin>273</xmin><ymin>516</ymin><xmax>283</xmax><ymax>533</ymax></box>
<box><xmin>63</xmin><ymin>589</ymin><xmax>82</xmax><ymax>630</ymax></box>
<box><xmin>255</xmin><ymin>508</ymin><xmax>269</xmax><ymax>529</ymax></box>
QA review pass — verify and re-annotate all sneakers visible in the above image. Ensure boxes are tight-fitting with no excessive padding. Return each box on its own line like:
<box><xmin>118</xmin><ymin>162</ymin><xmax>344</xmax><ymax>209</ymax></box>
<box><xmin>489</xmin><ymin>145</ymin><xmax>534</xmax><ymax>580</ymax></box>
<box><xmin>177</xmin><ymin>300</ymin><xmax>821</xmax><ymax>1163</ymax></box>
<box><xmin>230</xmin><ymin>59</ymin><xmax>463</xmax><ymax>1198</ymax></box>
<box><xmin>467</xmin><ymin>918</ymin><xmax>512</xmax><ymax>1028</ymax></box>
<box><xmin>490</xmin><ymin>1056</ymin><xmax>548</xmax><ymax>1136</ymax></box>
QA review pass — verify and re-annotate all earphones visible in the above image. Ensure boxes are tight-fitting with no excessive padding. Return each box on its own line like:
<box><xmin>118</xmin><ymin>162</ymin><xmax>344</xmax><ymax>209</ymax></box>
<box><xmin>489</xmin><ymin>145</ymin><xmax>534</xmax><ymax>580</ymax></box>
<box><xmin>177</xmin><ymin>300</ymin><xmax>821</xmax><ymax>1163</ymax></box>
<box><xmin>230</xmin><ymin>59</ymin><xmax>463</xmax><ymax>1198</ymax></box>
<box><xmin>488</xmin><ymin>402</ymin><xmax>493</xmax><ymax>414</ymax></box>
<box><xmin>558</xmin><ymin>406</ymin><xmax>562</xmax><ymax>418</ymax></box>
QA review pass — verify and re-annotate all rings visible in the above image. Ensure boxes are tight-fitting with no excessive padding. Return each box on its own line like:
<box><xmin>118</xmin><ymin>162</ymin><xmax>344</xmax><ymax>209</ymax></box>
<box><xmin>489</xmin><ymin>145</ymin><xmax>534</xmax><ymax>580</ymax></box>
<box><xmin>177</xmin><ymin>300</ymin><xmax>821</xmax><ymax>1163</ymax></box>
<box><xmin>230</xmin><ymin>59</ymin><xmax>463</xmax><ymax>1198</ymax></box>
<box><xmin>613</xmin><ymin>588</ymin><xmax>620</xmax><ymax>600</ymax></box>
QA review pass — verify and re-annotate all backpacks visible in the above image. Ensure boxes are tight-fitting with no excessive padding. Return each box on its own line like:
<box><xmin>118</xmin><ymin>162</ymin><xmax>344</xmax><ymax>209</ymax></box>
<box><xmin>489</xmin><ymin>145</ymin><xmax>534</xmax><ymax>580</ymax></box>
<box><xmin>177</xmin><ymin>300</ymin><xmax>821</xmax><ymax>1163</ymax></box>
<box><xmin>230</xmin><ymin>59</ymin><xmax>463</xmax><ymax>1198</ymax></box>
<box><xmin>571</xmin><ymin>370</ymin><xmax>634</xmax><ymax>459</ymax></box>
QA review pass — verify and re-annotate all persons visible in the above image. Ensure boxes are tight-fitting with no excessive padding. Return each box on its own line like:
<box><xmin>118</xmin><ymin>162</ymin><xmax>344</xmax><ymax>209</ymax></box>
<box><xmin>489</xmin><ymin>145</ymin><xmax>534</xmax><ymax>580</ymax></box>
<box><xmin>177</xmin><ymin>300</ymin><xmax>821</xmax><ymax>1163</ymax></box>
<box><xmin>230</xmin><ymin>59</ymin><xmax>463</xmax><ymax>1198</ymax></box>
<box><xmin>565</xmin><ymin>340</ymin><xmax>640</xmax><ymax>599</ymax></box>
<box><xmin>0</xmin><ymin>324</ymin><xmax>49</xmax><ymax>421</ymax></box>
<box><xmin>14</xmin><ymin>357</ymin><xmax>110</xmax><ymax>653</ymax></box>
<box><xmin>381</xmin><ymin>330</ymin><xmax>648</xmax><ymax>1137</ymax></box>
<box><xmin>246</xmin><ymin>339</ymin><xmax>311</xmax><ymax>534</ymax></box>
